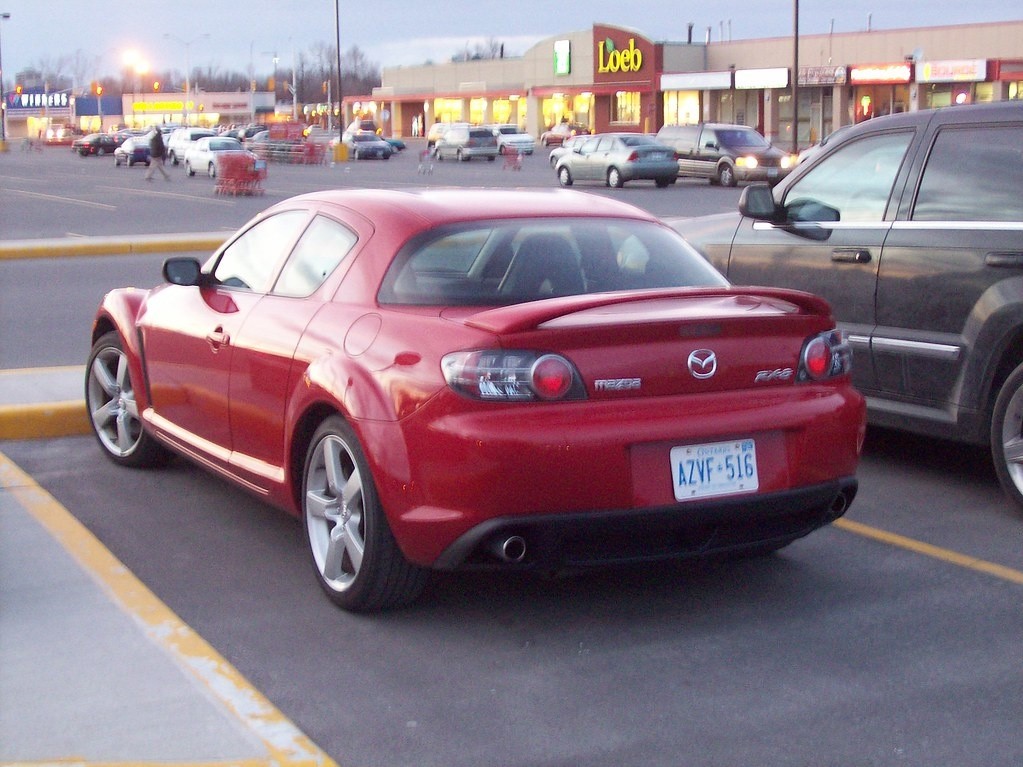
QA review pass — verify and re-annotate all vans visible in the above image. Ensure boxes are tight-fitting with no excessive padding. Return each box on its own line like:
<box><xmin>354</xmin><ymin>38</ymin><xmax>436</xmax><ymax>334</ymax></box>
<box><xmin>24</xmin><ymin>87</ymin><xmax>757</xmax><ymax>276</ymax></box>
<box><xmin>167</xmin><ymin>129</ymin><xmax>216</xmax><ymax>164</ymax></box>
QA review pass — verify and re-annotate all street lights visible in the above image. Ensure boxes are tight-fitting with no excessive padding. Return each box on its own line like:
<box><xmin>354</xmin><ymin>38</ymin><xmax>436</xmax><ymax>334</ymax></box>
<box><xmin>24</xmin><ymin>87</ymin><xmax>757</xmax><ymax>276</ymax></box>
<box><xmin>0</xmin><ymin>12</ymin><xmax>11</xmax><ymax>145</ymax></box>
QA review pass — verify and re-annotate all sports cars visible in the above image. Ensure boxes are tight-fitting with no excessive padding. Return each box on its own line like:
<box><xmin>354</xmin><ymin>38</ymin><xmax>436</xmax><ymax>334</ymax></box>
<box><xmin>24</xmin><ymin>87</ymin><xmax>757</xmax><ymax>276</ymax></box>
<box><xmin>82</xmin><ymin>186</ymin><xmax>872</xmax><ymax>615</ymax></box>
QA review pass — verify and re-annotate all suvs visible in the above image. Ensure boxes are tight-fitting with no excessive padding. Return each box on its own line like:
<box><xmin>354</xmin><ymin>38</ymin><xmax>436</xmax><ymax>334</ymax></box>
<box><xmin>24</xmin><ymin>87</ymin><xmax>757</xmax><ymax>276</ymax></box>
<box><xmin>435</xmin><ymin>126</ymin><xmax>499</xmax><ymax>163</ymax></box>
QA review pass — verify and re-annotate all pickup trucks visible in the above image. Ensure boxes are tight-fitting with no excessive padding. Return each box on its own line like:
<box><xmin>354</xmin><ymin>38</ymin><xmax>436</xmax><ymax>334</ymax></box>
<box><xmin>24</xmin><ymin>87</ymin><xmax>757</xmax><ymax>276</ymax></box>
<box><xmin>651</xmin><ymin>120</ymin><xmax>804</xmax><ymax>188</ymax></box>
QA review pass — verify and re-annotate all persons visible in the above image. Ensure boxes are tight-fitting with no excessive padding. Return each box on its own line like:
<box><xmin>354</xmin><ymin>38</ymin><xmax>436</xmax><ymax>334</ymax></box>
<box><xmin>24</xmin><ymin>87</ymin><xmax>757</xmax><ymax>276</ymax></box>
<box><xmin>146</xmin><ymin>125</ymin><xmax>170</xmax><ymax>181</ymax></box>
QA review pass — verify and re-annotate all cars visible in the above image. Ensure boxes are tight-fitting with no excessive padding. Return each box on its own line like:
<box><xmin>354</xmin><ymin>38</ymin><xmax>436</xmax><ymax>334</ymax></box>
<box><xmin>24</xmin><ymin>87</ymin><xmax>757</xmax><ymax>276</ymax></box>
<box><xmin>427</xmin><ymin>124</ymin><xmax>445</xmax><ymax>148</ymax></box>
<box><xmin>487</xmin><ymin>123</ymin><xmax>535</xmax><ymax>154</ymax></box>
<box><xmin>633</xmin><ymin>99</ymin><xmax>1023</xmax><ymax>512</ymax></box>
<box><xmin>380</xmin><ymin>138</ymin><xmax>407</xmax><ymax>154</ymax></box>
<box><xmin>347</xmin><ymin>131</ymin><xmax>392</xmax><ymax>160</ymax></box>
<box><xmin>112</xmin><ymin>136</ymin><xmax>154</xmax><ymax>168</ymax></box>
<box><xmin>548</xmin><ymin>134</ymin><xmax>594</xmax><ymax>169</ymax></box>
<box><xmin>185</xmin><ymin>136</ymin><xmax>258</xmax><ymax>178</ymax></box>
<box><xmin>215</xmin><ymin>122</ymin><xmax>327</xmax><ymax>164</ymax></box>
<box><xmin>72</xmin><ymin>132</ymin><xmax>118</xmax><ymax>156</ymax></box>
<box><xmin>113</xmin><ymin>126</ymin><xmax>180</xmax><ymax>143</ymax></box>
<box><xmin>555</xmin><ymin>132</ymin><xmax>680</xmax><ymax>189</ymax></box>
<box><xmin>541</xmin><ymin>123</ymin><xmax>591</xmax><ymax>148</ymax></box>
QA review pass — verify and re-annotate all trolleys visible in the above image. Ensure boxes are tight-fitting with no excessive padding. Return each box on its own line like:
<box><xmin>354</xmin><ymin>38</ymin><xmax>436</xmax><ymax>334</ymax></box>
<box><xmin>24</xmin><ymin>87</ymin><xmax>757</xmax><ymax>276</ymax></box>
<box><xmin>502</xmin><ymin>146</ymin><xmax>525</xmax><ymax>171</ymax></box>
<box><xmin>216</xmin><ymin>155</ymin><xmax>268</xmax><ymax>197</ymax></box>
<box><xmin>415</xmin><ymin>147</ymin><xmax>438</xmax><ymax>176</ymax></box>
